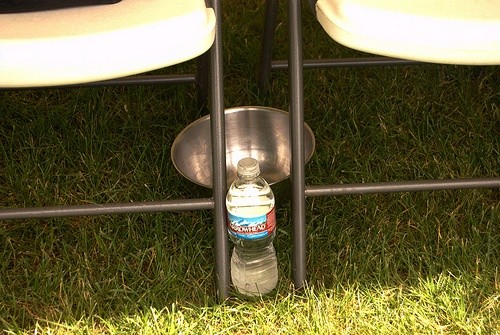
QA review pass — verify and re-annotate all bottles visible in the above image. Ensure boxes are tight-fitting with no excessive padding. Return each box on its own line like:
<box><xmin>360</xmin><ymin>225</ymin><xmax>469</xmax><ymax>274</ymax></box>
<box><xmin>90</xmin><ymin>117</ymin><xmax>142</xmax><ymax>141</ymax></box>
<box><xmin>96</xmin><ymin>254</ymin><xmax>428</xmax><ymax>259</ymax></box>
<box><xmin>225</xmin><ymin>158</ymin><xmax>278</xmax><ymax>297</ymax></box>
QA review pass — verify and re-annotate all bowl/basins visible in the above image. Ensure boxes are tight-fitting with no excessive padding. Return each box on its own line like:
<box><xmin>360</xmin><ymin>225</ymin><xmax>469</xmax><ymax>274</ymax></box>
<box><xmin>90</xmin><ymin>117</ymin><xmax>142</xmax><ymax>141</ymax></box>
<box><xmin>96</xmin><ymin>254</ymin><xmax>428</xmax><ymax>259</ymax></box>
<box><xmin>170</xmin><ymin>106</ymin><xmax>316</xmax><ymax>196</ymax></box>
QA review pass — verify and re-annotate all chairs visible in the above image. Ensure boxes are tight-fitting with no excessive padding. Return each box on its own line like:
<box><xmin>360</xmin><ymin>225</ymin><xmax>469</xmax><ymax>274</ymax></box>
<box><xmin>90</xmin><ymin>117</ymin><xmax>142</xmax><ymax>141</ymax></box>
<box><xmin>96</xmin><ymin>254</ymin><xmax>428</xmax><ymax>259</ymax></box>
<box><xmin>0</xmin><ymin>0</ymin><xmax>229</xmax><ymax>307</ymax></box>
<box><xmin>263</xmin><ymin>0</ymin><xmax>498</xmax><ymax>296</ymax></box>
